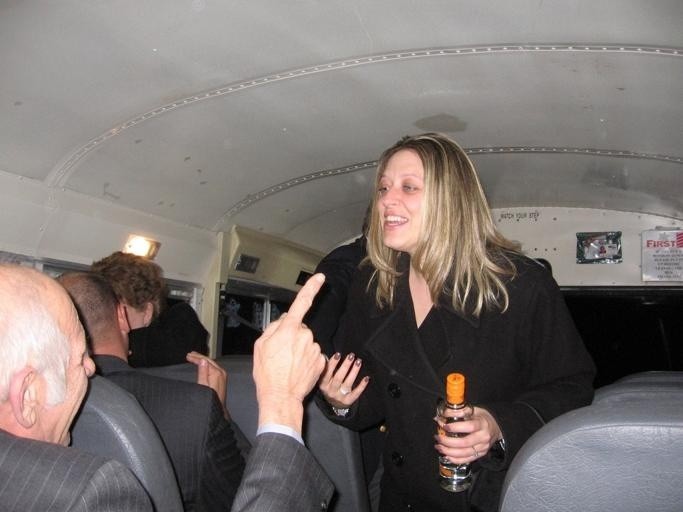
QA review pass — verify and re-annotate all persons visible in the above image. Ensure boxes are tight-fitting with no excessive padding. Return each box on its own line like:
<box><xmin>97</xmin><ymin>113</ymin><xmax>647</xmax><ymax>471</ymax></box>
<box><xmin>305</xmin><ymin>133</ymin><xmax>599</xmax><ymax>512</ymax></box>
<box><xmin>302</xmin><ymin>202</ymin><xmax>380</xmax><ymax>355</ymax></box>
<box><xmin>55</xmin><ymin>268</ymin><xmax>245</xmax><ymax>512</ymax></box>
<box><xmin>92</xmin><ymin>251</ymin><xmax>210</xmax><ymax>367</ymax></box>
<box><xmin>0</xmin><ymin>264</ymin><xmax>336</xmax><ymax>511</ymax></box>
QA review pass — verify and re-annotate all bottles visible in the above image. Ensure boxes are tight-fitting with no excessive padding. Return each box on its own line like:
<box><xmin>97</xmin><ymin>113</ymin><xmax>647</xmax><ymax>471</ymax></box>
<box><xmin>438</xmin><ymin>373</ymin><xmax>473</xmax><ymax>492</ymax></box>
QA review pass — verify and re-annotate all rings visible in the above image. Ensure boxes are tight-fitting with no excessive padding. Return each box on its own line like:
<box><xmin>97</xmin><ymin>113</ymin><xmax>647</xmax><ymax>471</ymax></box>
<box><xmin>340</xmin><ymin>386</ymin><xmax>351</xmax><ymax>396</ymax></box>
<box><xmin>472</xmin><ymin>446</ymin><xmax>479</xmax><ymax>457</ymax></box>
